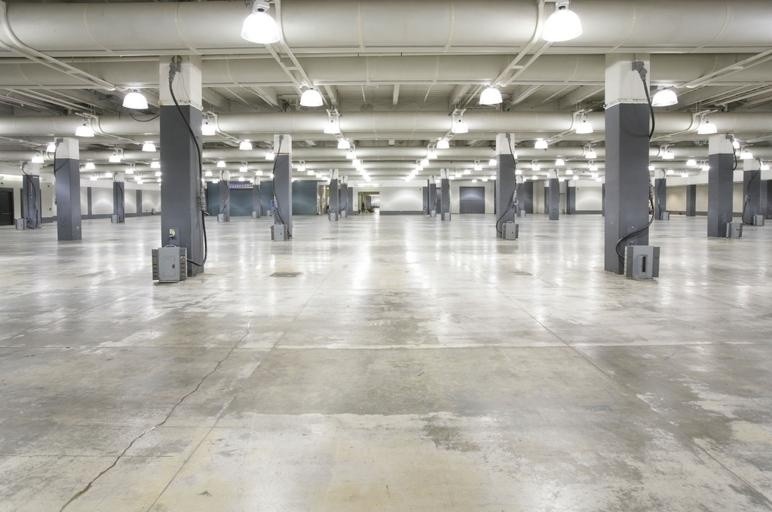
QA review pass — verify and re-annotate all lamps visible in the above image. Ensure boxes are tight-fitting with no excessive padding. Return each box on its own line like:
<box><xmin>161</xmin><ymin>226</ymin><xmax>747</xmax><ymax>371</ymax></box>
<box><xmin>651</xmin><ymin>87</ymin><xmax>679</xmax><ymax>107</ymax></box>
<box><xmin>515</xmin><ymin>138</ymin><xmax>605</xmax><ymax>186</ymax></box>
<box><xmin>648</xmin><ymin>142</ymin><xmax>709</xmax><ymax>186</ymax></box>
<box><xmin>452</xmin><ymin>118</ymin><xmax>468</xmax><ymax>134</ymax></box>
<box><xmin>242</xmin><ymin>1</ymin><xmax>283</xmax><ymax>44</ymax></box>
<box><xmin>324</xmin><ymin>120</ymin><xmax>340</xmax><ymax>133</ymax></box>
<box><xmin>202</xmin><ymin>140</ymin><xmax>274</xmax><ymax>185</ymax></box>
<box><xmin>32</xmin><ymin>140</ymin><xmax>163</xmax><ymax>188</ymax></box>
<box><xmin>122</xmin><ymin>89</ymin><xmax>148</xmax><ymax>109</ymax></box>
<box><xmin>299</xmin><ymin>86</ymin><xmax>322</xmax><ymax>106</ymax></box>
<box><xmin>542</xmin><ymin>3</ymin><xmax>582</xmax><ymax>43</ymax></box>
<box><xmin>576</xmin><ymin>117</ymin><xmax>594</xmax><ymax>135</ymax></box>
<box><xmin>696</xmin><ymin>115</ymin><xmax>717</xmax><ymax>135</ymax></box>
<box><xmin>733</xmin><ymin>138</ymin><xmax>768</xmax><ymax>178</ymax></box>
<box><xmin>75</xmin><ymin>123</ymin><xmax>94</xmax><ymax>136</ymax></box>
<box><xmin>479</xmin><ymin>85</ymin><xmax>503</xmax><ymax>106</ymax></box>
<box><xmin>201</xmin><ymin>122</ymin><xmax>214</xmax><ymax>136</ymax></box>
<box><xmin>291</xmin><ymin>138</ymin><xmax>372</xmax><ymax>187</ymax></box>
<box><xmin>402</xmin><ymin>140</ymin><xmax>497</xmax><ymax>185</ymax></box>
